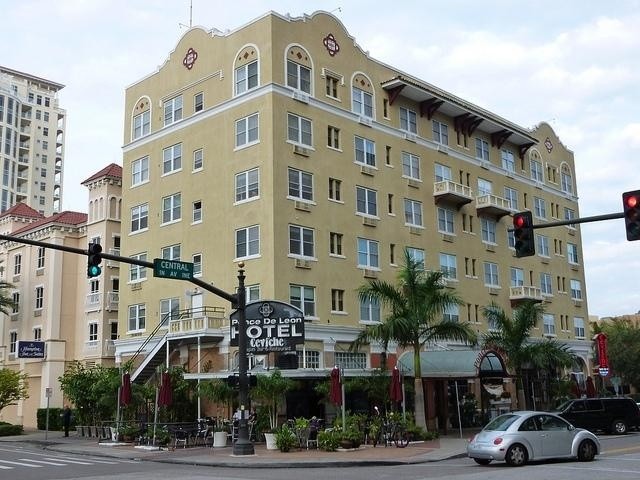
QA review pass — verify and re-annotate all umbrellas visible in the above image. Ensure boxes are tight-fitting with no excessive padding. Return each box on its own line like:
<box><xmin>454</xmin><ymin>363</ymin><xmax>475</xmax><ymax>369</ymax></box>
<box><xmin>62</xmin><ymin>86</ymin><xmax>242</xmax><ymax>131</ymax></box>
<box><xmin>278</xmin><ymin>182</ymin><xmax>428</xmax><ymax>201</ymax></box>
<box><xmin>153</xmin><ymin>368</ymin><xmax>172</xmax><ymax>445</ymax></box>
<box><xmin>116</xmin><ymin>369</ymin><xmax>132</xmax><ymax>429</ymax></box>
<box><xmin>390</xmin><ymin>365</ymin><xmax>406</xmax><ymax>422</ymax></box>
<box><xmin>330</xmin><ymin>364</ymin><xmax>346</xmax><ymax>433</ymax></box>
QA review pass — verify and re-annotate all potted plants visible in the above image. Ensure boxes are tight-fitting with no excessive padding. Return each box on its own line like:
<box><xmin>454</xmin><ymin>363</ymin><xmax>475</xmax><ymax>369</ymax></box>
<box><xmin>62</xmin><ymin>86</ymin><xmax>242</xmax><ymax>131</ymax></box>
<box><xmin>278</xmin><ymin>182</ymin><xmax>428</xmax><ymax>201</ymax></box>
<box><xmin>118</xmin><ymin>426</ymin><xmax>142</xmax><ymax>443</ymax></box>
<box><xmin>265</xmin><ymin>426</ymin><xmax>282</xmax><ymax>451</ymax></box>
<box><xmin>213</xmin><ymin>427</ymin><xmax>229</xmax><ymax>447</ymax></box>
<box><xmin>340</xmin><ymin>427</ymin><xmax>362</xmax><ymax>449</ymax></box>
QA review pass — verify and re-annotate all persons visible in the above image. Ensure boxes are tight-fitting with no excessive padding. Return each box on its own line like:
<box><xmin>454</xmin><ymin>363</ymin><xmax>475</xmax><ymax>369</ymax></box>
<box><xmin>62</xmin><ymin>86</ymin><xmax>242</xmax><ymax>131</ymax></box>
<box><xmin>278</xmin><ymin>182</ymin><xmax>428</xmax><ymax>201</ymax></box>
<box><xmin>248</xmin><ymin>407</ymin><xmax>257</xmax><ymax>426</ymax></box>
<box><xmin>60</xmin><ymin>404</ymin><xmax>71</xmax><ymax>437</ymax></box>
<box><xmin>233</xmin><ymin>407</ymin><xmax>241</xmax><ymax>426</ymax></box>
<box><xmin>308</xmin><ymin>415</ymin><xmax>321</xmax><ymax>441</ymax></box>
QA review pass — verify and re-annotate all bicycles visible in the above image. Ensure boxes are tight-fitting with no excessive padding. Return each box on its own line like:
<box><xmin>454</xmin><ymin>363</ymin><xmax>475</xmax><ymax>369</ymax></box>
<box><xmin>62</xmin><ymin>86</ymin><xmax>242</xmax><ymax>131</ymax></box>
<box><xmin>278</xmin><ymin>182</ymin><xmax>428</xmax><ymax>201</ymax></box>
<box><xmin>374</xmin><ymin>407</ymin><xmax>408</xmax><ymax>448</ymax></box>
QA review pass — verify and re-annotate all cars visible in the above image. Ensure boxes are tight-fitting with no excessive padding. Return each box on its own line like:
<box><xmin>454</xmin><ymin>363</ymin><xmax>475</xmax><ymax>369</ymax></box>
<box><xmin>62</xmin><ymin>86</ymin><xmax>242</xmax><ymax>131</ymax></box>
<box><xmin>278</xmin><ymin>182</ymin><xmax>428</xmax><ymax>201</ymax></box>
<box><xmin>467</xmin><ymin>412</ymin><xmax>600</xmax><ymax>466</ymax></box>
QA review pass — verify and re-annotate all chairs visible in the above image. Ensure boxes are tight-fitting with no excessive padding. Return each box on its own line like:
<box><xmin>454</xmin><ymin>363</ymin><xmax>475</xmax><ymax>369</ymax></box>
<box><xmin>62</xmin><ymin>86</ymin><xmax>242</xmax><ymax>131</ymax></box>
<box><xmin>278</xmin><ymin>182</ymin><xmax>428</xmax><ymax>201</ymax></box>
<box><xmin>168</xmin><ymin>417</ymin><xmax>215</xmax><ymax>450</ymax></box>
<box><xmin>288</xmin><ymin>416</ymin><xmax>324</xmax><ymax>452</ymax></box>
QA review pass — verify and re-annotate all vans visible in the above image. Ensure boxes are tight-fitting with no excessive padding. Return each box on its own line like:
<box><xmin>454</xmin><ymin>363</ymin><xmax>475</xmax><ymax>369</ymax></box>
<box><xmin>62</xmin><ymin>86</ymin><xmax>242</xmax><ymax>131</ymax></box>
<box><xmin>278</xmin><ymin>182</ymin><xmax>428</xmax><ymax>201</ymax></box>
<box><xmin>542</xmin><ymin>398</ymin><xmax>639</xmax><ymax>434</ymax></box>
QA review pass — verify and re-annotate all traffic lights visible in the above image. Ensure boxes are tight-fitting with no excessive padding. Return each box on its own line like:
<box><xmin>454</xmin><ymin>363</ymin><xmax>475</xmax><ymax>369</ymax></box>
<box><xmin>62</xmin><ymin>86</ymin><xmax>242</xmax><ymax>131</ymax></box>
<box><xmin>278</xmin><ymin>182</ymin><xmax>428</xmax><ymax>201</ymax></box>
<box><xmin>87</xmin><ymin>243</ymin><xmax>101</xmax><ymax>277</ymax></box>
<box><xmin>622</xmin><ymin>191</ymin><xmax>640</xmax><ymax>241</ymax></box>
<box><xmin>513</xmin><ymin>211</ymin><xmax>535</xmax><ymax>256</ymax></box>
